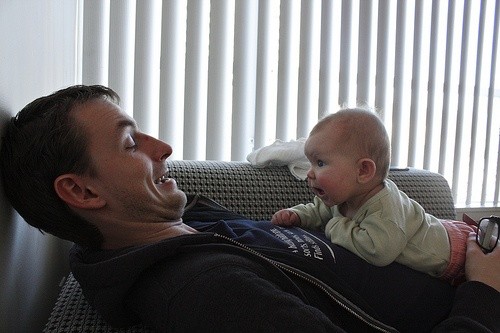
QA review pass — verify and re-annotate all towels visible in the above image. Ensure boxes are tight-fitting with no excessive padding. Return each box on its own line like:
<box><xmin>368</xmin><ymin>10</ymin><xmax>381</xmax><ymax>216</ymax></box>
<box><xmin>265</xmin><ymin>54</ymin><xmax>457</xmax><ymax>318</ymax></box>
<box><xmin>246</xmin><ymin>137</ymin><xmax>312</xmax><ymax>181</ymax></box>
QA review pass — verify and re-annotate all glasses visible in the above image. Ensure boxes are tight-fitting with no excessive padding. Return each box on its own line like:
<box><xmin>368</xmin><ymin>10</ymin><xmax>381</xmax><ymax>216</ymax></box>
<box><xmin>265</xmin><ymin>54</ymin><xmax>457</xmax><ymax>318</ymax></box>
<box><xmin>463</xmin><ymin>213</ymin><xmax>500</xmax><ymax>253</ymax></box>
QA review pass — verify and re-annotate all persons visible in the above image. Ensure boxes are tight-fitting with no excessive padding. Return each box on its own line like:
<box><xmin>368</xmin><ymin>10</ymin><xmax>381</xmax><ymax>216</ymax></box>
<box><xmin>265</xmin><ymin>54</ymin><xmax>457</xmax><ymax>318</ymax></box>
<box><xmin>0</xmin><ymin>85</ymin><xmax>500</xmax><ymax>333</ymax></box>
<box><xmin>271</xmin><ymin>108</ymin><xmax>488</xmax><ymax>286</ymax></box>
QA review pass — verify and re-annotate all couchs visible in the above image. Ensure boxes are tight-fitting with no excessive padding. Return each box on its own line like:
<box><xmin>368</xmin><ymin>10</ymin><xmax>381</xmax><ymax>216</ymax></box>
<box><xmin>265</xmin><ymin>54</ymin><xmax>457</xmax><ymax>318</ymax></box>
<box><xmin>40</xmin><ymin>160</ymin><xmax>459</xmax><ymax>333</ymax></box>
